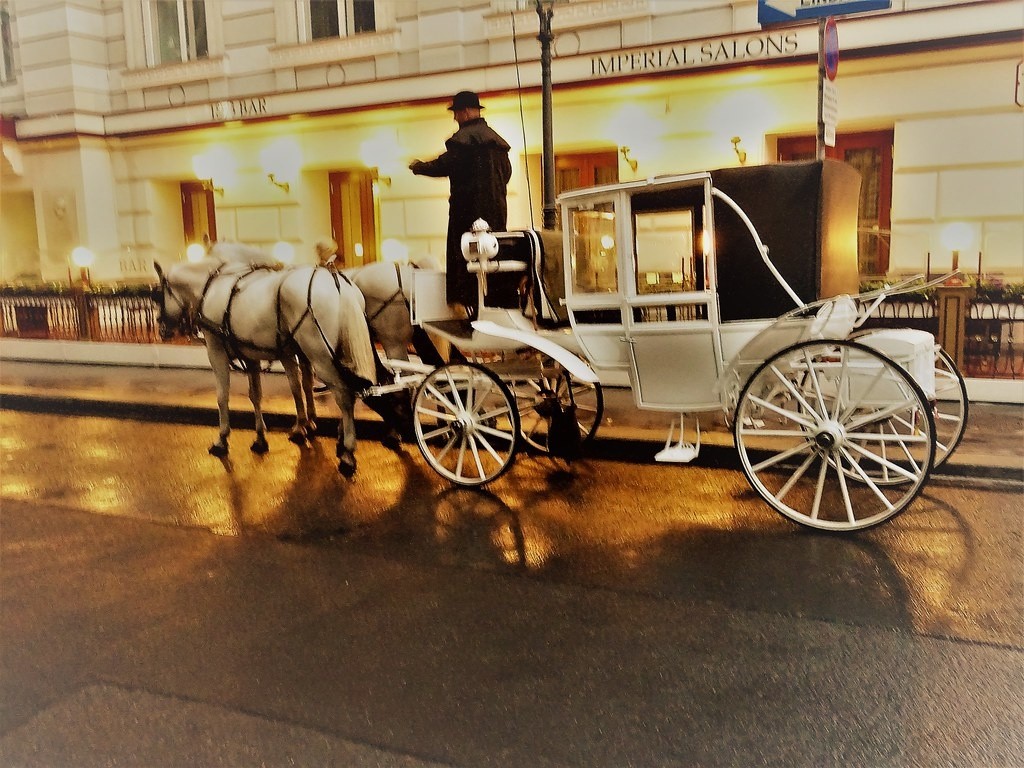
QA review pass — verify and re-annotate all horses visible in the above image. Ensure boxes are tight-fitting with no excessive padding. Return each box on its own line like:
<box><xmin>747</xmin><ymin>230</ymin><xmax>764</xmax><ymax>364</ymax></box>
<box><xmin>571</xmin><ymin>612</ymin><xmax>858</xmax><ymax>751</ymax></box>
<box><xmin>150</xmin><ymin>242</ymin><xmax>451</xmax><ymax>481</ymax></box>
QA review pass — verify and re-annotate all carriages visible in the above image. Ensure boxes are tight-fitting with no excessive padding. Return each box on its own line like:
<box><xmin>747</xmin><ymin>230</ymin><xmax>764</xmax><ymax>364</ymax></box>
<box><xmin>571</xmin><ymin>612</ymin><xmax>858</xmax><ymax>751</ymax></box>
<box><xmin>153</xmin><ymin>157</ymin><xmax>972</xmax><ymax>534</ymax></box>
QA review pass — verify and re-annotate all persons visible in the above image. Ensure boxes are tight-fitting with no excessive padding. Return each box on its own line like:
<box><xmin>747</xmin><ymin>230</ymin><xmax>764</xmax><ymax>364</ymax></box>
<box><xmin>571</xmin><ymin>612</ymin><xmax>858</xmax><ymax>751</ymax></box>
<box><xmin>408</xmin><ymin>89</ymin><xmax>514</xmax><ymax>330</ymax></box>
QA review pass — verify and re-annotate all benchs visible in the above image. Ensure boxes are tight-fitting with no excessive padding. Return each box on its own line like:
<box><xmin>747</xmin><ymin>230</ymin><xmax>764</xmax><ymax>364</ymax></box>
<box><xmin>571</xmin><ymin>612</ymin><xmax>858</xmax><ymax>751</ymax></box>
<box><xmin>439</xmin><ymin>233</ymin><xmax>547</xmax><ymax>335</ymax></box>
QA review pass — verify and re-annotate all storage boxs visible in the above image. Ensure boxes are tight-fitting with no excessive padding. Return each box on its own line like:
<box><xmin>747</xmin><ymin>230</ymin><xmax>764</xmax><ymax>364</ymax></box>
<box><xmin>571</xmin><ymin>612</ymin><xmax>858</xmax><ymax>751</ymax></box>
<box><xmin>847</xmin><ymin>329</ymin><xmax>933</xmax><ymax>402</ymax></box>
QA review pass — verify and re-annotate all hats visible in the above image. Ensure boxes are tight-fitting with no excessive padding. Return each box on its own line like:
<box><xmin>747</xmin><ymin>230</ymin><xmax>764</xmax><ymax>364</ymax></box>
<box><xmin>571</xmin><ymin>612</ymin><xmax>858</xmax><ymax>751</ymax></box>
<box><xmin>448</xmin><ymin>92</ymin><xmax>485</xmax><ymax>109</ymax></box>
